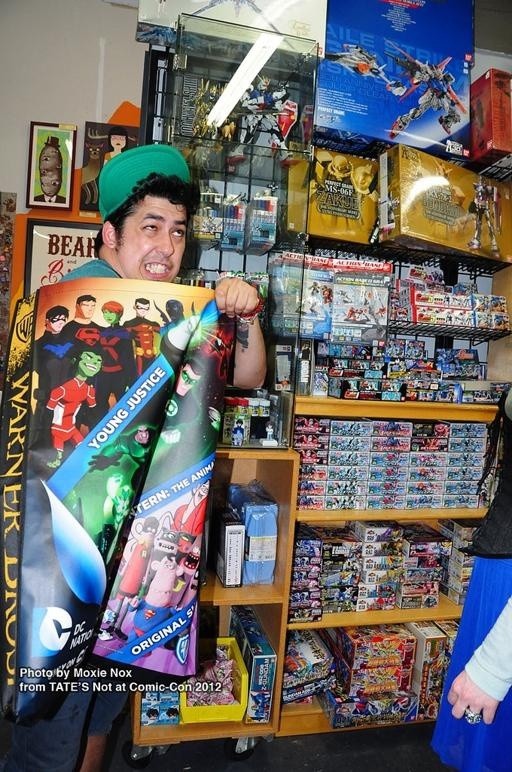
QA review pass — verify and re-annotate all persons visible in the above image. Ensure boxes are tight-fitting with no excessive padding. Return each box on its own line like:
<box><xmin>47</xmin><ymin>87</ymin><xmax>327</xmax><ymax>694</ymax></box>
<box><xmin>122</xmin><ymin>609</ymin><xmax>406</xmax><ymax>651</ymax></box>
<box><xmin>428</xmin><ymin>382</ymin><xmax>512</xmax><ymax>772</ymax></box>
<box><xmin>140</xmin><ymin>0</ymin><xmax>511</xmax><ymax>729</ymax></box>
<box><xmin>1</xmin><ymin>143</ymin><xmax>271</xmax><ymax>770</ymax></box>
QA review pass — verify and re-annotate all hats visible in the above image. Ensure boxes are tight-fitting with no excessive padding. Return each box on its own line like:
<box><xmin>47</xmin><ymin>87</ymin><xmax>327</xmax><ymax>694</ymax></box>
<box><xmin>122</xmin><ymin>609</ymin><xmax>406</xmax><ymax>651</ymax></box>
<box><xmin>98</xmin><ymin>144</ymin><xmax>192</xmax><ymax>221</ymax></box>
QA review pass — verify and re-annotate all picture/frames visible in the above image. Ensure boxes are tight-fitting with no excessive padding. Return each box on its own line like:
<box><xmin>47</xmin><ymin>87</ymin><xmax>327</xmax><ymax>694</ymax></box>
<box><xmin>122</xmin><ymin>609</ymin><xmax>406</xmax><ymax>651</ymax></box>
<box><xmin>25</xmin><ymin>119</ymin><xmax>78</xmax><ymax>211</ymax></box>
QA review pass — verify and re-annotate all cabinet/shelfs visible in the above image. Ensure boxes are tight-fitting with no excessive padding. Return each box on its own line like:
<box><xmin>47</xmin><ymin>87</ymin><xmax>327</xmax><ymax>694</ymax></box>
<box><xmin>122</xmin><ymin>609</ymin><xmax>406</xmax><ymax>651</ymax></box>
<box><xmin>130</xmin><ymin>445</ymin><xmax>301</xmax><ymax>747</ymax></box>
<box><xmin>279</xmin><ymin>393</ymin><xmax>499</xmax><ymax>736</ymax></box>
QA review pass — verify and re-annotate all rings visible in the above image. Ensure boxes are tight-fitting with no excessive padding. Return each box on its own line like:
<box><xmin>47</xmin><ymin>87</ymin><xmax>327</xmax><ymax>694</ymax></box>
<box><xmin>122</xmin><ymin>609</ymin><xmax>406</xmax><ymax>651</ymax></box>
<box><xmin>463</xmin><ymin>707</ymin><xmax>482</xmax><ymax>725</ymax></box>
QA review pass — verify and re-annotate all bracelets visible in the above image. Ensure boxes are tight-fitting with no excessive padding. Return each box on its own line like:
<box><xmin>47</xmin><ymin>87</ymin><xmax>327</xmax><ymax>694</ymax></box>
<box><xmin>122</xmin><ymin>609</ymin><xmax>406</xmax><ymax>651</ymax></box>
<box><xmin>234</xmin><ymin>283</ymin><xmax>263</xmax><ymax>326</ymax></box>
